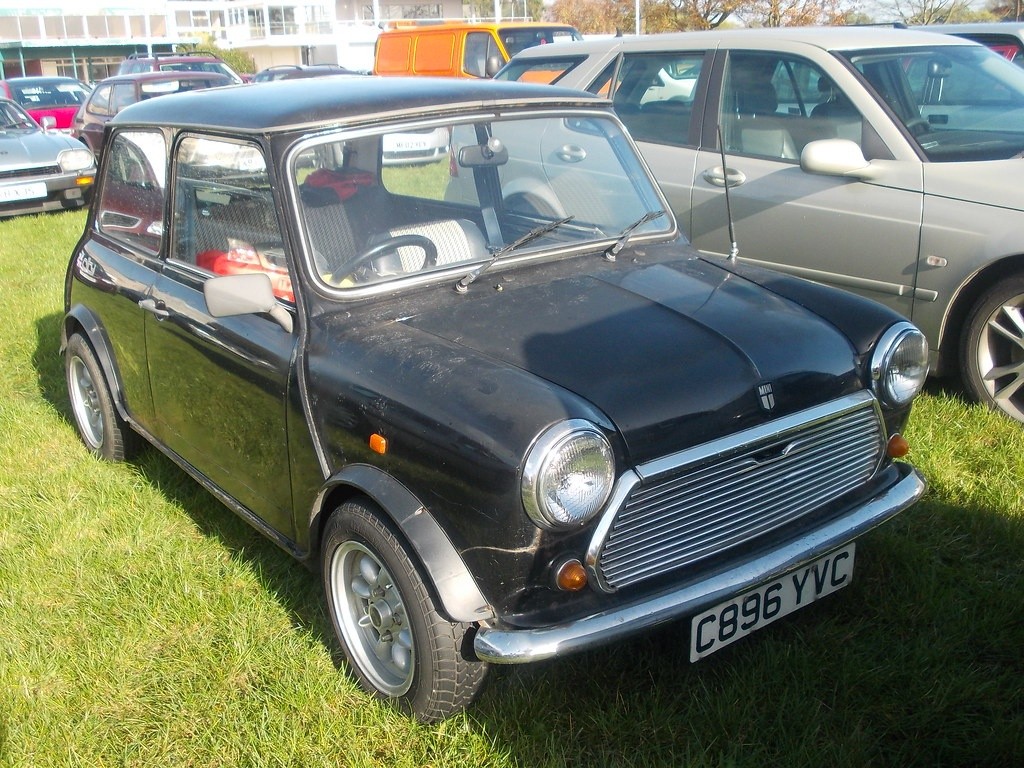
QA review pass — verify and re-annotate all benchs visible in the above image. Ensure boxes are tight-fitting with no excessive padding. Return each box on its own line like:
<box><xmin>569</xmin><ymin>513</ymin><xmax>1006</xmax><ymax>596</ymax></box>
<box><xmin>183</xmin><ymin>186</ymin><xmax>357</xmax><ymax>285</ymax></box>
<box><xmin>641</xmin><ymin>99</ymin><xmax>689</xmax><ymax>143</ymax></box>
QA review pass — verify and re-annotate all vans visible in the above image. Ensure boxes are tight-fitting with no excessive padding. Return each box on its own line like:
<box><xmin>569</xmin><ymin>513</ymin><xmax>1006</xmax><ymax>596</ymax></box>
<box><xmin>371</xmin><ymin>16</ymin><xmax>622</xmax><ymax>100</ymax></box>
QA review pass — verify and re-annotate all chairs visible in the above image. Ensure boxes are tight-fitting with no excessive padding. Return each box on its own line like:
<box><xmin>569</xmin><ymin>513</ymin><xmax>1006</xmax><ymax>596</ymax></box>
<box><xmin>737</xmin><ymin>78</ymin><xmax>795</xmax><ymax>158</ymax></box>
<box><xmin>370</xmin><ymin>217</ymin><xmax>492</xmax><ymax>279</ymax></box>
<box><xmin>810</xmin><ymin>74</ymin><xmax>862</xmax><ymax>154</ymax></box>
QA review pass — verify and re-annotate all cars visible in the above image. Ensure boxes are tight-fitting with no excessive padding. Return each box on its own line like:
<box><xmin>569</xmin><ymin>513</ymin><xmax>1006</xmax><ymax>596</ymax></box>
<box><xmin>771</xmin><ymin>22</ymin><xmax>1023</xmax><ymax>133</ymax></box>
<box><xmin>441</xmin><ymin>25</ymin><xmax>1024</xmax><ymax>424</ymax></box>
<box><xmin>553</xmin><ymin>34</ymin><xmax>698</xmax><ymax>109</ymax></box>
<box><xmin>117</xmin><ymin>51</ymin><xmax>244</xmax><ymax>85</ymax></box>
<box><xmin>641</xmin><ymin>63</ymin><xmax>697</xmax><ymax>102</ymax></box>
<box><xmin>68</xmin><ymin>70</ymin><xmax>236</xmax><ymax>164</ymax></box>
<box><xmin>0</xmin><ymin>97</ymin><xmax>98</xmax><ymax>220</ymax></box>
<box><xmin>0</xmin><ymin>76</ymin><xmax>119</xmax><ymax>138</ymax></box>
<box><xmin>56</xmin><ymin>75</ymin><xmax>932</xmax><ymax>727</ymax></box>
<box><xmin>247</xmin><ymin>64</ymin><xmax>366</xmax><ymax>83</ymax></box>
<box><xmin>78</xmin><ymin>113</ymin><xmax>164</xmax><ymax>253</ymax></box>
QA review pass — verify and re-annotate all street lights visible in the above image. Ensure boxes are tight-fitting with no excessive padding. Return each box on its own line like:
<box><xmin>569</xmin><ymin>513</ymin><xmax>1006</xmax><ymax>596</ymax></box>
<box><xmin>307</xmin><ymin>47</ymin><xmax>316</xmax><ymax>66</ymax></box>
